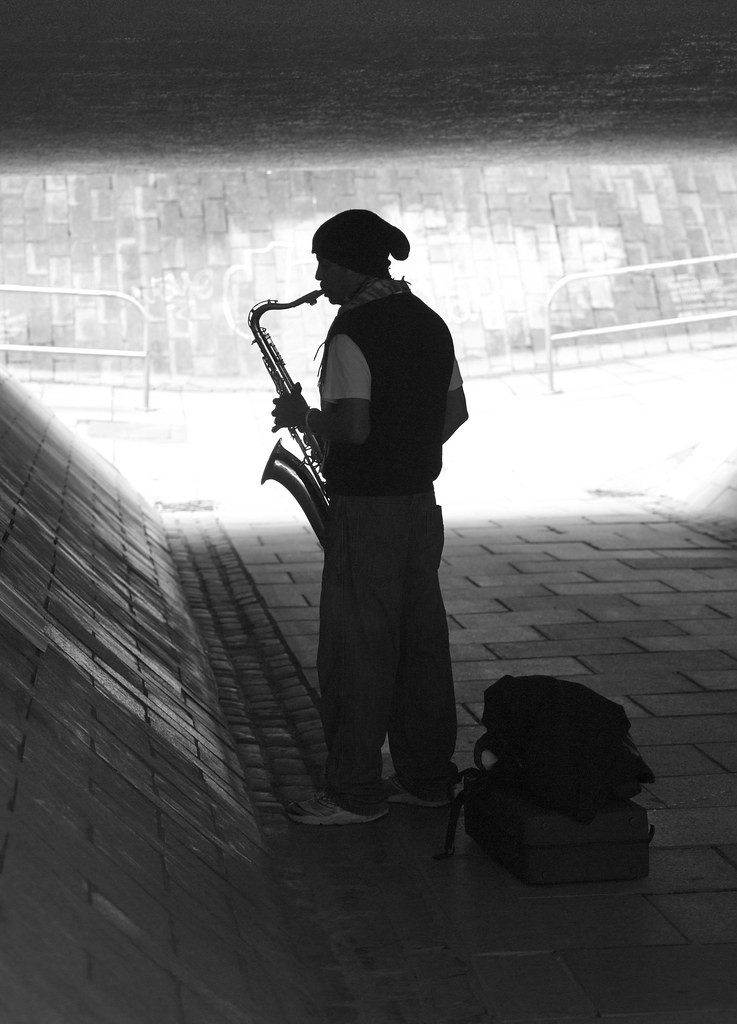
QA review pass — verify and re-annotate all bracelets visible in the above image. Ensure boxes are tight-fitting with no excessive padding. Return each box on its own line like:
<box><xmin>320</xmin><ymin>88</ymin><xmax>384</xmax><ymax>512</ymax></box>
<box><xmin>305</xmin><ymin>408</ymin><xmax>321</xmax><ymax>433</ymax></box>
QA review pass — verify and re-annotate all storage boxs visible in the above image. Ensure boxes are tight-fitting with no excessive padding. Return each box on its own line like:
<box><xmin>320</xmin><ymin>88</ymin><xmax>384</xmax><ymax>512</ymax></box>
<box><xmin>461</xmin><ymin>777</ymin><xmax>651</xmax><ymax>887</ymax></box>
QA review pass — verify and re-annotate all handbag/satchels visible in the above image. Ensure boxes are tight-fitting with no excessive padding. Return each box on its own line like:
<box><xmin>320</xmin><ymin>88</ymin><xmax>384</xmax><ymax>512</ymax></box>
<box><xmin>473</xmin><ymin>673</ymin><xmax>655</xmax><ymax>825</ymax></box>
<box><xmin>444</xmin><ymin>760</ymin><xmax>657</xmax><ymax>885</ymax></box>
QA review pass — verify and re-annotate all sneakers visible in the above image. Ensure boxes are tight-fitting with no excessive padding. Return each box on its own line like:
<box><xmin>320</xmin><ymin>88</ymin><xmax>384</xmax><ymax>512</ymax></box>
<box><xmin>381</xmin><ymin>773</ymin><xmax>455</xmax><ymax>807</ymax></box>
<box><xmin>283</xmin><ymin>787</ymin><xmax>389</xmax><ymax>825</ymax></box>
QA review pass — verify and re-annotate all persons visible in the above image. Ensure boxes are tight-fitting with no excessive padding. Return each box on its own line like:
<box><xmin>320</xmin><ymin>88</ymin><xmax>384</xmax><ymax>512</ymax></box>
<box><xmin>272</xmin><ymin>209</ymin><xmax>469</xmax><ymax>825</ymax></box>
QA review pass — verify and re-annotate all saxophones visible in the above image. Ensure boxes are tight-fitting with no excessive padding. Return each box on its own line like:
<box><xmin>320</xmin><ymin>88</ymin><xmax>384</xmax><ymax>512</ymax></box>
<box><xmin>244</xmin><ymin>289</ymin><xmax>334</xmax><ymax>554</ymax></box>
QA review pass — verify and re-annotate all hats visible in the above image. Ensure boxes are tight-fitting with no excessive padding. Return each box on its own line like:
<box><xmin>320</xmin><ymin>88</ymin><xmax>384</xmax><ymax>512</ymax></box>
<box><xmin>311</xmin><ymin>209</ymin><xmax>410</xmax><ymax>276</ymax></box>
